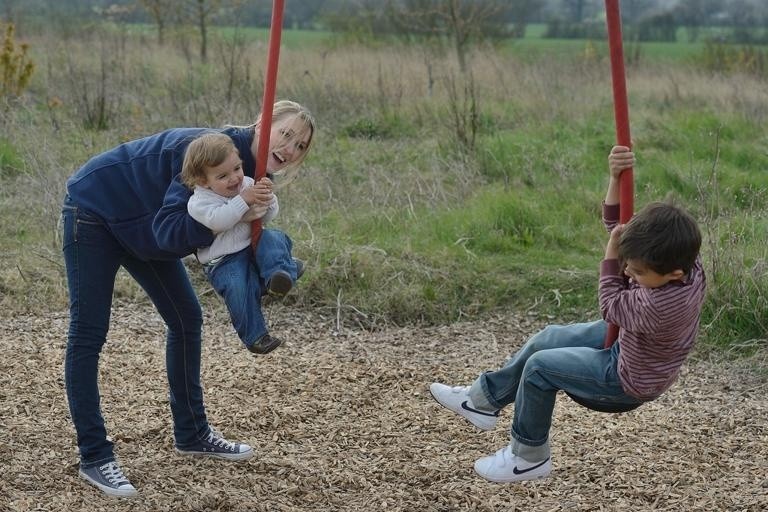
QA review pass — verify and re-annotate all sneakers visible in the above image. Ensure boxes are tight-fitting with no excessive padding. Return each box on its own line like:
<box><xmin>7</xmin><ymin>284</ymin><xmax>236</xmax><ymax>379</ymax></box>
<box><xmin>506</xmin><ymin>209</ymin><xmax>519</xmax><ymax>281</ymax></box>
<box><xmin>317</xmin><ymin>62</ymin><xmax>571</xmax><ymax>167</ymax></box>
<box><xmin>473</xmin><ymin>445</ymin><xmax>551</xmax><ymax>483</ymax></box>
<box><xmin>429</xmin><ymin>382</ymin><xmax>499</xmax><ymax>431</ymax></box>
<box><xmin>246</xmin><ymin>334</ymin><xmax>281</xmax><ymax>354</ymax></box>
<box><xmin>175</xmin><ymin>426</ymin><xmax>253</xmax><ymax>461</ymax></box>
<box><xmin>266</xmin><ymin>270</ymin><xmax>294</xmax><ymax>301</ymax></box>
<box><xmin>79</xmin><ymin>457</ymin><xmax>137</xmax><ymax>497</ymax></box>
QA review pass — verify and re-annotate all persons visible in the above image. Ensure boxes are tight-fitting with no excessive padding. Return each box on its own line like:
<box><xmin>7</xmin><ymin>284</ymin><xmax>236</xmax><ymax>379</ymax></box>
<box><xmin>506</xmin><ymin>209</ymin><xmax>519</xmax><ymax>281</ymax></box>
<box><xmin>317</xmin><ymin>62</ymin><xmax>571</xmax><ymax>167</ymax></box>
<box><xmin>181</xmin><ymin>133</ymin><xmax>299</xmax><ymax>353</ymax></box>
<box><xmin>62</xmin><ymin>99</ymin><xmax>318</xmax><ymax>496</ymax></box>
<box><xmin>429</xmin><ymin>144</ymin><xmax>707</xmax><ymax>483</ymax></box>
